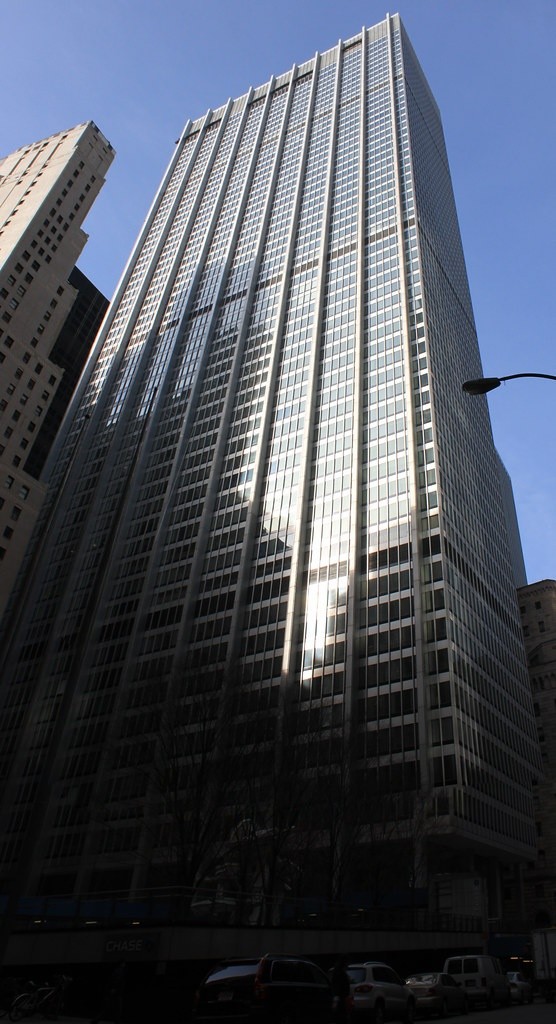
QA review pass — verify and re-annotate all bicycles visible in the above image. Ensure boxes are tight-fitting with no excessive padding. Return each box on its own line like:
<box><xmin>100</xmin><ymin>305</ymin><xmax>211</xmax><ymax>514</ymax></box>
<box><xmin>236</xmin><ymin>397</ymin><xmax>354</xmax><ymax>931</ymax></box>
<box><xmin>0</xmin><ymin>972</ymin><xmax>68</xmax><ymax>1022</ymax></box>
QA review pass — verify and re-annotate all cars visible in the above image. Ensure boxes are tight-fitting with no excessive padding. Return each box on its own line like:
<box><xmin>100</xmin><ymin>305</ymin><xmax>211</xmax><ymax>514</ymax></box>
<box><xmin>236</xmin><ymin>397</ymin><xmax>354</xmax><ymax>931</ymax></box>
<box><xmin>404</xmin><ymin>972</ymin><xmax>469</xmax><ymax>1020</ymax></box>
<box><xmin>507</xmin><ymin>972</ymin><xmax>533</xmax><ymax>1005</ymax></box>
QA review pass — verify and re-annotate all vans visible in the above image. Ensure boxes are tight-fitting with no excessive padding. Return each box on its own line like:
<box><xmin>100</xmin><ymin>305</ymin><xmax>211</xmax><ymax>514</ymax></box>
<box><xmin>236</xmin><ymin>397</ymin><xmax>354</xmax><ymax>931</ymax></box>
<box><xmin>443</xmin><ymin>955</ymin><xmax>511</xmax><ymax>1011</ymax></box>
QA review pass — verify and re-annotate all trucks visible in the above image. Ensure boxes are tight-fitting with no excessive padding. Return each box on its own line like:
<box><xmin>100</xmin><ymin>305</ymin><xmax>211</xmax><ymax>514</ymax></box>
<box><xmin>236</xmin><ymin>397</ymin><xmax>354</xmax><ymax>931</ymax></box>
<box><xmin>531</xmin><ymin>927</ymin><xmax>556</xmax><ymax>1004</ymax></box>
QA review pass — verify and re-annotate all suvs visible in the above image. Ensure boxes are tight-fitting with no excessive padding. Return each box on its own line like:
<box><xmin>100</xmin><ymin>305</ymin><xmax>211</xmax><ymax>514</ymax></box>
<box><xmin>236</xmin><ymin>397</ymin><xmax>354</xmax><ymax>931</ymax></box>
<box><xmin>192</xmin><ymin>951</ymin><xmax>356</xmax><ymax>1024</ymax></box>
<box><xmin>345</xmin><ymin>961</ymin><xmax>417</xmax><ymax>1024</ymax></box>
